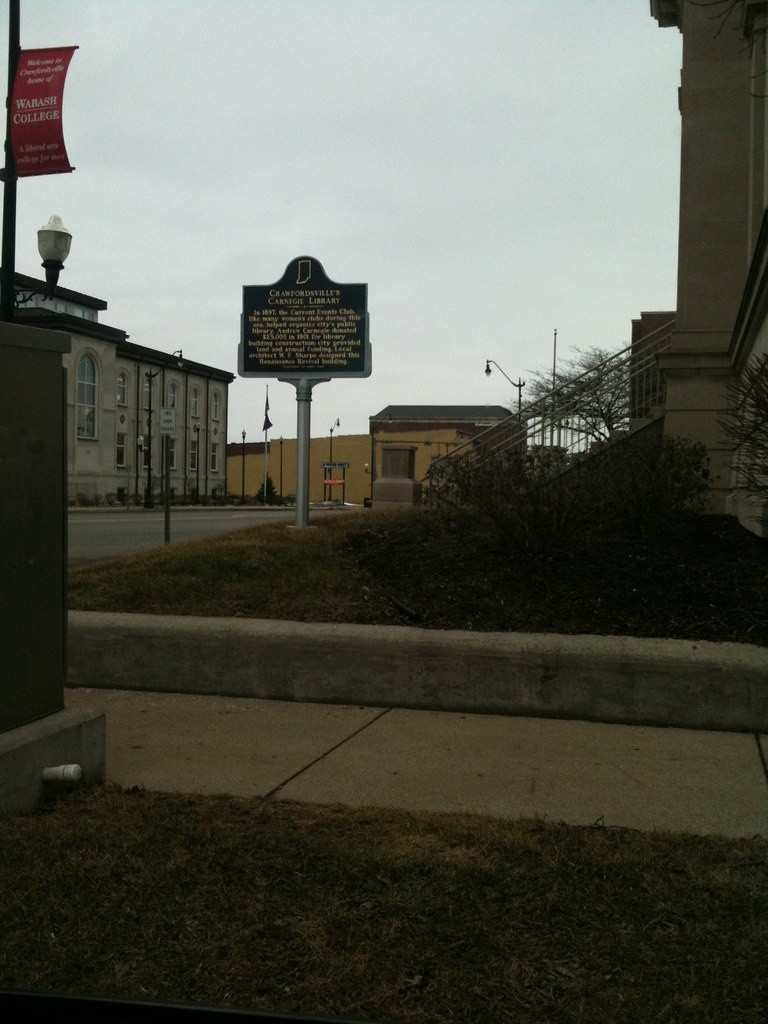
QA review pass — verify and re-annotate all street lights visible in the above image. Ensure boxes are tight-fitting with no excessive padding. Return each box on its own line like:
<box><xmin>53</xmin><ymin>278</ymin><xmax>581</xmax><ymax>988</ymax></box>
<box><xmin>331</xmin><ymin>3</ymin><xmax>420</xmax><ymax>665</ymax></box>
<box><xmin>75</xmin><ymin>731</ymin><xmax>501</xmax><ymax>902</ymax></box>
<box><xmin>279</xmin><ymin>436</ymin><xmax>287</xmax><ymax>506</ymax></box>
<box><xmin>241</xmin><ymin>430</ymin><xmax>246</xmax><ymax>504</ymax></box>
<box><xmin>365</xmin><ymin>413</ymin><xmax>393</xmax><ymax>500</ymax></box>
<box><xmin>328</xmin><ymin>419</ymin><xmax>340</xmax><ymax>500</ymax></box>
<box><xmin>135</xmin><ymin>350</ymin><xmax>183</xmax><ymax>509</ymax></box>
<box><xmin>485</xmin><ymin>360</ymin><xmax>525</xmax><ymax>453</ymax></box>
